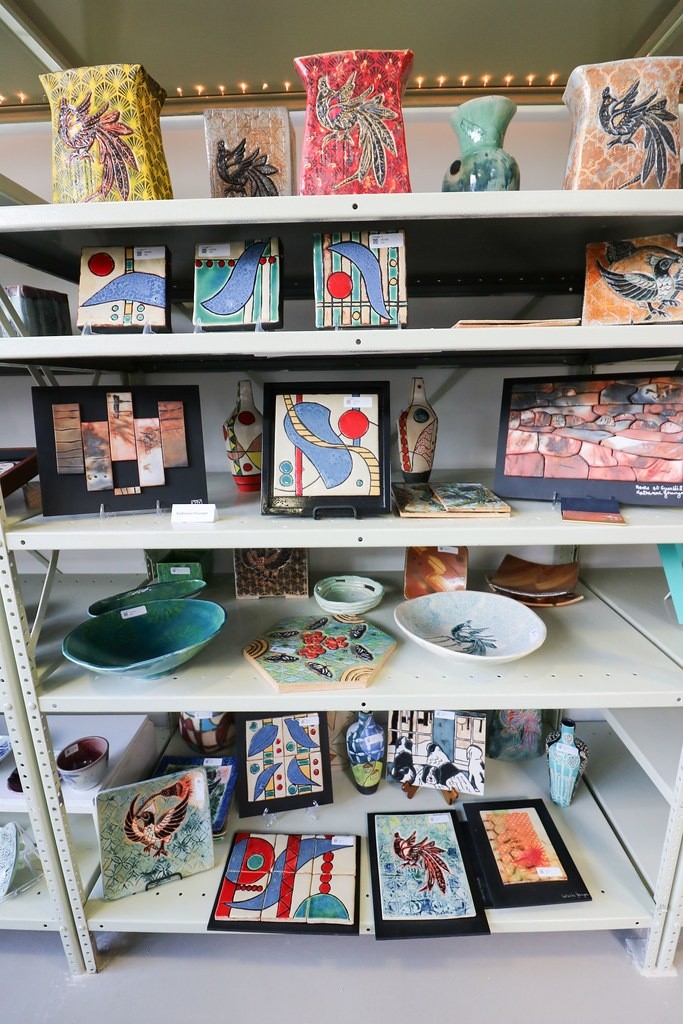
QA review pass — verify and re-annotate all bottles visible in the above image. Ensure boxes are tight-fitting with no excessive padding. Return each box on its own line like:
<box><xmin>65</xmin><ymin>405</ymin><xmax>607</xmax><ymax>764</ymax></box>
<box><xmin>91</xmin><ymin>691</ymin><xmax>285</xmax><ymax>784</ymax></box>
<box><xmin>345</xmin><ymin>709</ymin><xmax>386</xmax><ymax>795</ymax></box>
<box><xmin>151</xmin><ymin>754</ymin><xmax>239</xmax><ymax>843</ymax></box>
<box><xmin>178</xmin><ymin>711</ymin><xmax>238</xmax><ymax>755</ymax></box>
<box><xmin>544</xmin><ymin>716</ymin><xmax>590</xmax><ymax>808</ymax></box>
<box><xmin>395</xmin><ymin>377</ymin><xmax>439</xmax><ymax>484</ymax></box>
<box><xmin>221</xmin><ymin>380</ymin><xmax>263</xmax><ymax>492</ymax></box>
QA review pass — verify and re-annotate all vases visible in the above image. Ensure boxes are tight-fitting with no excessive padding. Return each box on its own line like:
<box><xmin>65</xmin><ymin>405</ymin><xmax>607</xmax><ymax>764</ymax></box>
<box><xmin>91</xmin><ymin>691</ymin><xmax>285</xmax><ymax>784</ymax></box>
<box><xmin>396</xmin><ymin>376</ymin><xmax>439</xmax><ymax>485</ymax></box>
<box><xmin>545</xmin><ymin>718</ymin><xmax>588</xmax><ymax>809</ymax></box>
<box><xmin>294</xmin><ymin>48</ymin><xmax>417</xmax><ymax>196</ymax></box>
<box><xmin>346</xmin><ymin>711</ymin><xmax>385</xmax><ymax>795</ymax></box>
<box><xmin>39</xmin><ymin>64</ymin><xmax>173</xmax><ymax>204</ymax></box>
<box><xmin>222</xmin><ymin>379</ymin><xmax>264</xmax><ymax>493</ymax></box>
<box><xmin>561</xmin><ymin>55</ymin><xmax>683</xmax><ymax>190</ymax></box>
<box><xmin>441</xmin><ymin>94</ymin><xmax>520</xmax><ymax>192</ymax></box>
<box><xmin>487</xmin><ymin>709</ymin><xmax>546</xmax><ymax>761</ymax></box>
<box><xmin>179</xmin><ymin>712</ymin><xmax>234</xmax><ymax>753</ymax></box>
<box><xmin>203</xmin><ymin>106</ymin><xmax>298</xmax><ymax>198</ymax></box>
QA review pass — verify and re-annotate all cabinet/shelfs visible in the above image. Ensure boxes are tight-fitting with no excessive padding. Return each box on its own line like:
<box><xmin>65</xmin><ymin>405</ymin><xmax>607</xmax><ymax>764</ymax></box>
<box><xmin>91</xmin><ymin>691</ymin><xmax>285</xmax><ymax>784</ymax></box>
<box><xmin>0</xmin><ymin>174</ymin><xmax>683</xmax><ymax>978</ymax></box>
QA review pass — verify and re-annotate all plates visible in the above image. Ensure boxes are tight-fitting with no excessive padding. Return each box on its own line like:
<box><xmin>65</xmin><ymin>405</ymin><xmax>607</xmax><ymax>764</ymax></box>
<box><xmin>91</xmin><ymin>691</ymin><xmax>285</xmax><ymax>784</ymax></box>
<box><xmin>0</xmin><ymin>734</ymin><xmax>12</xmax><ymax>762</ymax></box>
<box><xmin>0</xmin><ymin>822</ymin><xmax>20</xmax><ymax>897</ymax></box>
<box><xmin>482</xmin><ymin>551</ymin><xmax>584</xmax><ymax>608</ymax></box>
<box><xmin>91</xmin><ymin>767</ymin><xmax>214</xmax><ymax>902</ymax></box>
<box><xmin>86</xmin><ymin>580</ymin><xmax>208</xmax><ymax>617</ymax></box>
<box><xmin>6</xmin><ymin>750</ymin><xmax>62</xmax><ymax>795</ymax></box>
<box><xmin>393</xmin><ymin>589</ymin><xmax>548</xmax><ymax>665</ymax></box>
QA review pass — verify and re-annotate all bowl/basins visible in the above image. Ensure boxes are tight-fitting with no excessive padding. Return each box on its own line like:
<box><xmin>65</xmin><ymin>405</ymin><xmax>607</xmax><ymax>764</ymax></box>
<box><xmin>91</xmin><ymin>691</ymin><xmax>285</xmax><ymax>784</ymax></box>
<box><xmin>54</xmin><ymin>736</ymin><xmax>110</xmax><ymax>792</ymax></box>
<box><xmin>60</xmin><ymin>598</ymin><xmax>226</xmax><ymax>681</ymax></box>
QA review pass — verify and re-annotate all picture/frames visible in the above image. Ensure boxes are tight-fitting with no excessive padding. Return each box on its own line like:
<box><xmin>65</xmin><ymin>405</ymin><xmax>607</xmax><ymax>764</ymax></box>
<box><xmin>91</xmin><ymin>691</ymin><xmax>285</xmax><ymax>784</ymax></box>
<box><xmin>233</xmin><ymin>711</ymin><xmax>333</xmax><ymax>818</ymax></box>
<box><xmin>261</xmin><ymin>382</ymin><xmax>392</xmax><ymax>516</ymax></box>
<box><xmin>491</xmin><ymin>369</ymin><xmax>683</xmax><ymax>508</ymax></box>
<box><xmin>32</xmin><ymin>386</ymin><xmax>208</xmax><ymax>516</ymax></box>
